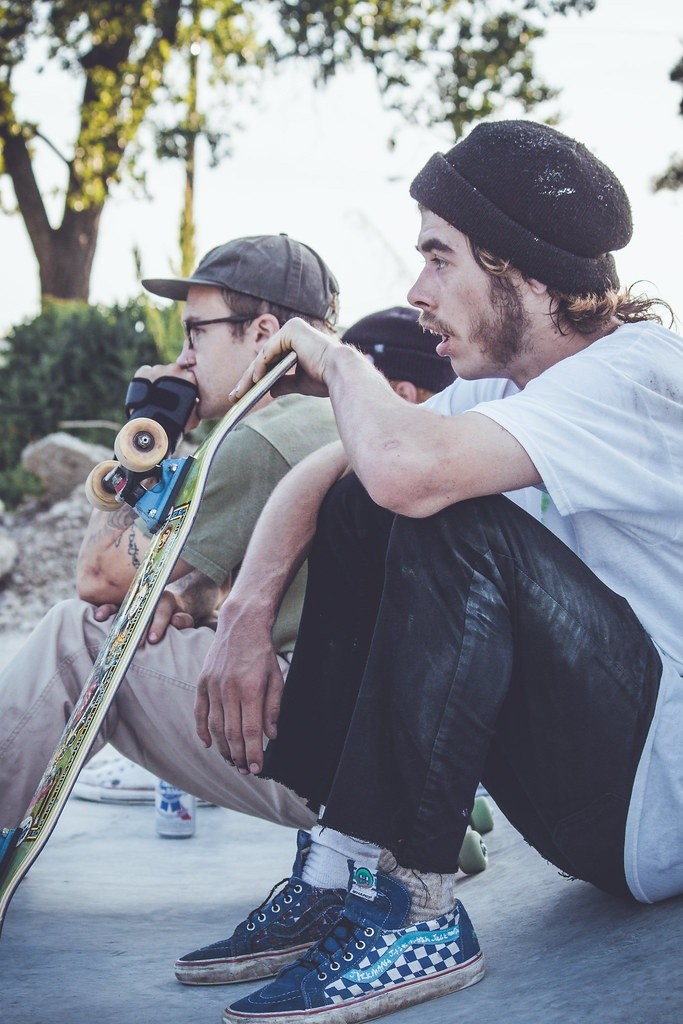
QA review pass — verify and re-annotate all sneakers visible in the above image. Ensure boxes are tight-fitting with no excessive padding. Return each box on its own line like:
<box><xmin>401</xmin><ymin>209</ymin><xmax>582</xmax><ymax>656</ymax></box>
<box><xmin>176</xmin><ymin>831</ymin><xmax>486</xmax><ymax>1024</ymax></box>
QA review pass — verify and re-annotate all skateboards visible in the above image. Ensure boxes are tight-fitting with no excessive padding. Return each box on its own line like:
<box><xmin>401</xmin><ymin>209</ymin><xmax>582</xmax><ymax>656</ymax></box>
<box><xmin>0</xmin><ymin>349</ymin><xmax>298</xmax><ymax>933</ymax></box>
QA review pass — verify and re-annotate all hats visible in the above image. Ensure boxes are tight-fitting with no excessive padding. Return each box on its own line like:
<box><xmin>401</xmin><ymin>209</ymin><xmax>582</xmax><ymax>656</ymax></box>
<box><xmin>409</xmin><ymin>121</ymin><xmax>632</xmax><ymax>300</ymax></box>
<box><xmin>141</xmin><ymin>234</ymin><xmax>340</xmax><ymax>333</ymax></box>
<box><xmin>340</xmin><ymin>307</ymin><xmax>458</xmax><ymax>394</ymax></box>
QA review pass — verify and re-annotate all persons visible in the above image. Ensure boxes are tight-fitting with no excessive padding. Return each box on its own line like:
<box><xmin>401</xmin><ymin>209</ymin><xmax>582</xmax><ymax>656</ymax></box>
<box><xmin>69</xmin><ymin>307</ymin><xmax>458</xmax><ymax>807</ymax></box>
<box><xmin>174</xmin><ymin>120</ymin><xmax>683</xmax><ymax>1024</ymax></box>
<box><xmin>0</xmin><ymin>233</ymin><xmax>340</xmax><ymax>839</ymax></box>
